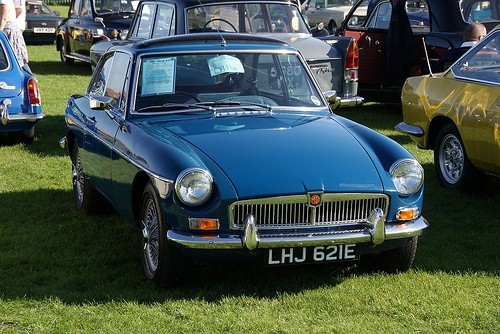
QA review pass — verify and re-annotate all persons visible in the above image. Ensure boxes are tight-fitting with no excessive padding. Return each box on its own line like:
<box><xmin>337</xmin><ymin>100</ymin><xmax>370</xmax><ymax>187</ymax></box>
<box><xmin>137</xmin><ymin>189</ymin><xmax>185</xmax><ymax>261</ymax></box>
<box><xmin>460</xmin><ymin>22</ymin><xmax>487</xmax><ymax>49</ymax></box>
<box><xmin>0</xmin><ymin>0</ymin><xmax>28</xmax><ymax>69</ymax></box>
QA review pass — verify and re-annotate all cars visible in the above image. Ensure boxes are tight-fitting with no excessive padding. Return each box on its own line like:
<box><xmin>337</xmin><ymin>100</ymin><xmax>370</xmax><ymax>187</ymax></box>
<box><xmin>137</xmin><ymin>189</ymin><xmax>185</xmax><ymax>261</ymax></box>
<box><xmin>55</xmin><ymin>0</ymin><xmax>170</xmax><ymax>67</ymax></box>
<box><xmin>334</xmin><ymin>1</ymin><xmax>500</xmax><ymax>113</ymax></box>
<box><xmin>89</xmin><ymin>1</ymin><xmax>364</xmax><ymax>112</ymax></box>
<box><xmin>58</xmin><ymin>31</ymin><xmax>430</xmax><ymax>283</ymax></box>
<box><xmin>0</xmin><ymin>29</ymin><xmax>46</xmax><ymax>145</ymax></box>
<box><xmin>22</xmin><ymin>0</ymin><xmax>66</xmax><ymax>44</ymax></box>
<box><xmin>248</xmin><ymin>0</ymin><xmax>431</xmax><ymax>34</ymax></box>
<box><xmin>394</xmin><ymin>22</ymin><xmax>500</xmax><ymax>191</ymax></box>
